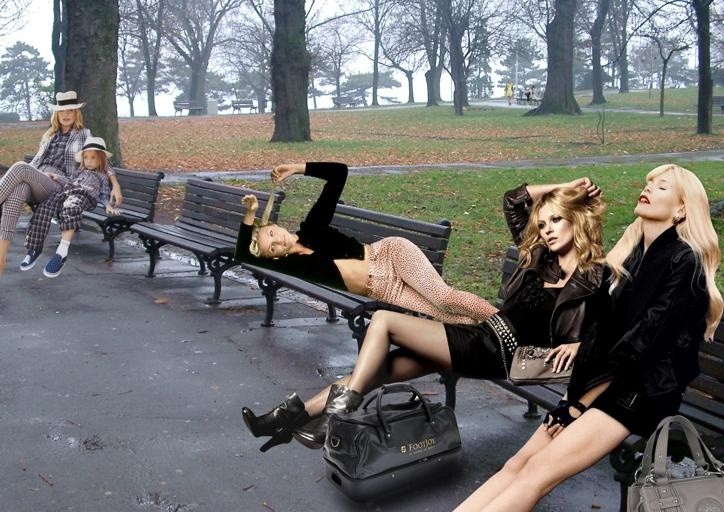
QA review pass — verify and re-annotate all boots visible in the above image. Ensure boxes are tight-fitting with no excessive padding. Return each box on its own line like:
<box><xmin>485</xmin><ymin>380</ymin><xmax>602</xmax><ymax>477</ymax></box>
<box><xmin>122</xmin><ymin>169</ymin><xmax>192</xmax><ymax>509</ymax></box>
<box><xmin>289</xmin><ymin>383</ymin><xmax>364</xmax><ymax>450</ymax></box>
<box><xmin>241</xmin><ymin>392</ymin><xmax>313</xmax><ymax>453</ymax></box>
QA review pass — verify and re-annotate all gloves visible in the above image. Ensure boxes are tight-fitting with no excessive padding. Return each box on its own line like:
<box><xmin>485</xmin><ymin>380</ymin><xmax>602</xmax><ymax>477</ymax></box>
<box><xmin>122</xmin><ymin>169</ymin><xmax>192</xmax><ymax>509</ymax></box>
<box><xmin>542</xmin><ymin>399</ymin><xmax>587</xmax><ymax>428</ymax></box>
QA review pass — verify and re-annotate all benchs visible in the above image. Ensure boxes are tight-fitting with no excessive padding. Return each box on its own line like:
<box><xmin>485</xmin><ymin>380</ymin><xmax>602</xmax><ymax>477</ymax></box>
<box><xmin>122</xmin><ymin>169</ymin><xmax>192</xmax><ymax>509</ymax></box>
<box><xmin>232</xmin><ymin>100</ymin><xmax>257</xmax><ymax>114</ymax></box>
<box><xmin>513</xmin><ymin>97</ymin><xmax>543</xmax><ymax>106</ymax></box>
<box><xmin>24</xmin><ymin>155</ymin><xmax>165</xmax><ymax>261</ymax></box>
<box><xmin>332</xmin><ymin>96</ymin><xmax>356</xmax><ymax>109</ymax></box>
<box><xmin>436</xmin><ymin>245</ymin><xmax>724</xmax><ymax>512</ymax></box>
<box><xmin>129</xmin><ymin>178</ymin><xmax>285</xmax><ymax>304</ymax></box>
<box><xmin>174</xmin><ymin>99</ymin><xmax>202</xmax><ymax>116</ymax></box>
<box><xmin>241</xmin><ymin>200</ymin><xmax>452</xmax><ymax>356</ymax></box>
<box><xmin>712</xmin><ymin>96</ymin><xmax>724</xmax><ymax>113</ymax></box>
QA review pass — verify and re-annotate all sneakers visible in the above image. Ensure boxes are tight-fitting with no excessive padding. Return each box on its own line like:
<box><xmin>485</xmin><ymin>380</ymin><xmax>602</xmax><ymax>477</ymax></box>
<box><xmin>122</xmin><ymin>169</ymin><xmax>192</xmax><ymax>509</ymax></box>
<box><xmin>43</xmin><ymin>250</ymin><xmax>68</xmax><ymax>278</ymax></box>
<box><xmin>19</xmin><ymin>247</ymin><xmax>42</xmax><ymax>271</ymax></box>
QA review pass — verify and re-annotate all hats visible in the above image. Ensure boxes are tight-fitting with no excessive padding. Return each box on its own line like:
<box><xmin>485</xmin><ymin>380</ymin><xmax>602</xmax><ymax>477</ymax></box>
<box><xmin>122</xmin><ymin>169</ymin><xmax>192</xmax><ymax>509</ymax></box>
<box><xmin>74</xmin><ymin>136</ymin><xmax>114</xmax><ymax>163</ymax></box>
<box><xmin>46</xmin><ymin>90</ymin><xmax>87</xmax><ymax>111</ymax></box>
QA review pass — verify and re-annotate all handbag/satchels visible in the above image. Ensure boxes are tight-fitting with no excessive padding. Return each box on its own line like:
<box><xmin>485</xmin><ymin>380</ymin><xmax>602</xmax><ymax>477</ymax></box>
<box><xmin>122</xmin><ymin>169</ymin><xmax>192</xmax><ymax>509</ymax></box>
<box><xmin>319</xmin><ymin>397</ymin><xmax>464</xmax><ymax>503</ymax></box>
<box><xmin>626</xmin><ymin>416</ymin><xmax>724</xmax><ymax>512</ymax></box>
<box><xmin>509</xmin><ymin>345</ymin><xmax>576</xmax><ymax>386</ymax></box>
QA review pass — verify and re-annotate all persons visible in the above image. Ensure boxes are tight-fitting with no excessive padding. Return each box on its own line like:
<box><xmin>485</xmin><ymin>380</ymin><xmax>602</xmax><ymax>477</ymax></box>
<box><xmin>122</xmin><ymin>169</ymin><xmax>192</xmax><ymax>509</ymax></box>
<box><xmin>453</xmin><ymin>163</ymin><xmax>723</xmax><ymax>512</ymax></box>
<box><xmin>0</xmin><ymin>90</ymin><xmax>123</xmax><ymax>277</ymax></box>
<box><xmin>524</xmin><ymin>84</ymin><xmax>536</xmax><ymax>106</ymax></box>
<box><xmin>235</xmin><ymin>161</ymin><xmax>502</xmax><ymax>326</ymax></box>
<box><xmin>503</xmin><ymin>78</ymin><xmax>515</xmax><ymax>106</ymax></box>
<box><xmin>241</xmin><ymin>177</ymin><xmax>614</xmax><ymax>453</ymax></box>
<box><xmin>18</xmin><ymin>136</ymin><xmax>122</xmax><ymax>277</ymax></box>
